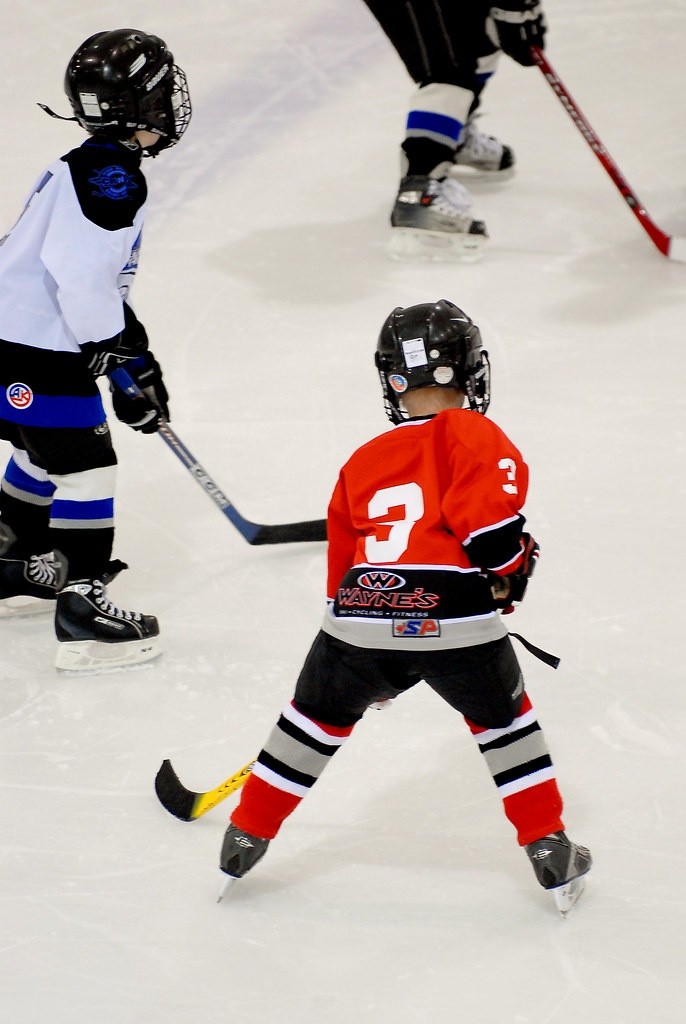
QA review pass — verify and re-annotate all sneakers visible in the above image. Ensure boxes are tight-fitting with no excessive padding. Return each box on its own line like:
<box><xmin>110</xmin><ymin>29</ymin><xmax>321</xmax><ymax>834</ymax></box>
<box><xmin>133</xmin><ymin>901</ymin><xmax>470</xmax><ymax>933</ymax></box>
<box><xmin>0</xmin><ymin>522</ymin><xmax>108</xmax><ymax>617</ymax></box>
<box><xmin>216</xmin><ymin>821</ymin><xmax>269</xmax><ymax>905</ymax></box>
<box><xmin>525</xmin><ymin>830</ymin><xmax>592</xmax><ymax>920</ymax></box>
<box><xmin>452</xmin><ymin>98</ymin><xmax>516</xmax><ymax>182</ymax></box>
<box><xmin>54</xmin><ymin>550</ymin><xmax>164</xmax><ymax>670</ymax></box>
<box><xmin>391</xmin><ymin>136</ymin><xmax>491</xmax><ymax>260</ymax></box>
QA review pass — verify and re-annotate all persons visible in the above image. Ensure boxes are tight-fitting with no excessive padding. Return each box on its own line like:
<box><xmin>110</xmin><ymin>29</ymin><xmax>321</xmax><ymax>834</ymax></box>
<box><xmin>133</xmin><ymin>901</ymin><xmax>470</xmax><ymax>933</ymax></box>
<box><xmin>215</xmin><ymin>300</ymin><xmax>594</xmax><ymax>922</ymax></box>
<box><xmin>364</xmin><ymin>0</ymin><xmax>549</xmax><ymax>262</ymax></box>
<box><xmin>0</xmin><ymin>27</ymin><xmax>193</xmax><ymax>672</ymax></box>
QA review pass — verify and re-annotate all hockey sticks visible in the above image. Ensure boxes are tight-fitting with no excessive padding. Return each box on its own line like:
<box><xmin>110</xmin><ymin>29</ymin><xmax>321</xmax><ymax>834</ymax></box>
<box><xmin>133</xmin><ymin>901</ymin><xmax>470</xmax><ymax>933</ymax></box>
<box><xmin>149</xmin><ymin>412</ymin><xmax>328</xmax><ymax>547</ymax></box>
<box><xmin>535</xmin><ymin>48</ymin><xmax>686</xmax><ymax>268</ymax></box>
<box><xmin>157</xmin><ymin>752</ymin><xmax>264</xmax><ymax>823</ymax></box>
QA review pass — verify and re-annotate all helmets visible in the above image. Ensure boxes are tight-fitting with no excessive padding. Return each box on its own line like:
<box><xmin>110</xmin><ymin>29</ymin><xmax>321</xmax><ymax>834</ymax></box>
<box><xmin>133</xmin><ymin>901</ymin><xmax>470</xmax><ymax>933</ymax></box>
<box><xmin>374</xmin><ymin>299</ymin><xmax>486</xmax><ymax>399</ymax></box>
<box><xmin>64</xmin><ymin>29</ymin><xmax>174</xmax><ymax>134</ymax></box>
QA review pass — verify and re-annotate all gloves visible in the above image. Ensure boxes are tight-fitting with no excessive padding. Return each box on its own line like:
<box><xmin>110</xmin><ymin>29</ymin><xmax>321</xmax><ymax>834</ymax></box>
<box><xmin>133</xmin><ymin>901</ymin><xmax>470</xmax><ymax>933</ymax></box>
<box><xmin>488</xmin><ymin>531</ymin><xmax>540</xmax><ymax>615</ymax></box>
<box><xmin>105</xmin><ymin>350</ymin><xmax>171</xmax><ymax>435</ymax></box>
<box><xmin>78</xmin><ymin>300</ymin><xmax>149</xmax><ymax>376</ymax></box>
<box><xmin>486</xmin><ymin>0</ymin><xmax>548</xmax><ymax>66</ymax></box>
